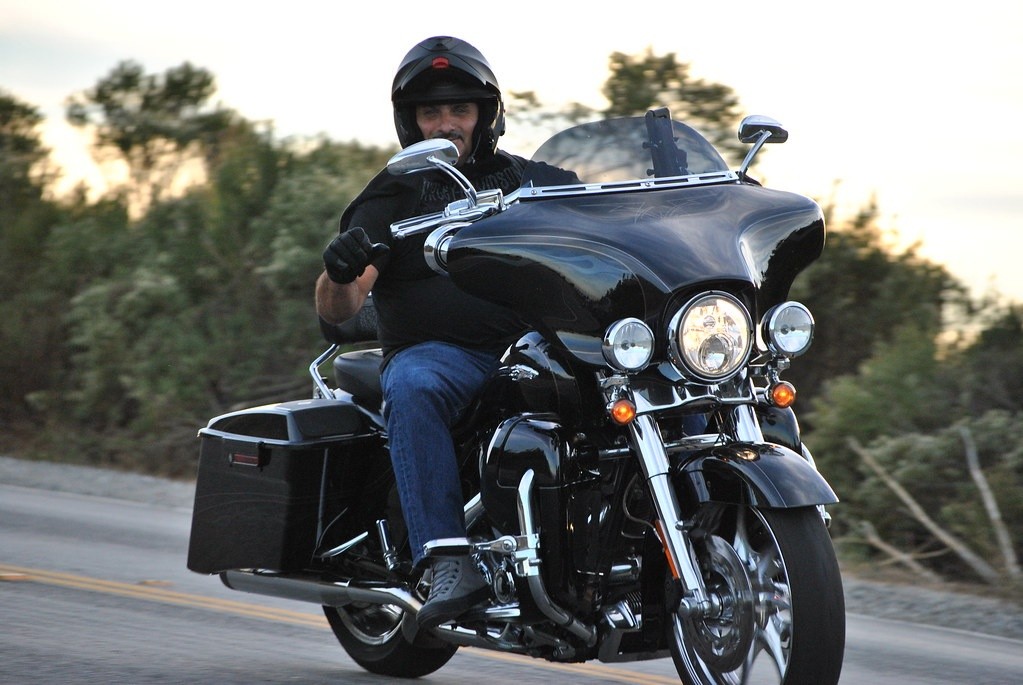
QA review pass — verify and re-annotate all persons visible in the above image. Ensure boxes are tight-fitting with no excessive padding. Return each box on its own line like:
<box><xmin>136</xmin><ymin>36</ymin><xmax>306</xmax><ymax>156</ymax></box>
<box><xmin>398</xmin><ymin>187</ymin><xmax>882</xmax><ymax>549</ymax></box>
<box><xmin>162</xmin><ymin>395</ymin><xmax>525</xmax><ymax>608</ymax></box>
<box><xmin>315</xmin><ymin>35</ymin><xmax>587</xmax><ymax>632</ymax></box>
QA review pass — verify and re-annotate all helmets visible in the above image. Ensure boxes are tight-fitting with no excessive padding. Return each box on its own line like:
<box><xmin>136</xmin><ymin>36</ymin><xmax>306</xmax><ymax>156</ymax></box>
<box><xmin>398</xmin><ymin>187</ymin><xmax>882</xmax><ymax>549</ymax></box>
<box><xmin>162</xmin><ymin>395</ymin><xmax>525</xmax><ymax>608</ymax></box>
<box><xmin>390</xmin><ymin>34</ymin><xmax>505</xmax><ymax>150</ymax></box>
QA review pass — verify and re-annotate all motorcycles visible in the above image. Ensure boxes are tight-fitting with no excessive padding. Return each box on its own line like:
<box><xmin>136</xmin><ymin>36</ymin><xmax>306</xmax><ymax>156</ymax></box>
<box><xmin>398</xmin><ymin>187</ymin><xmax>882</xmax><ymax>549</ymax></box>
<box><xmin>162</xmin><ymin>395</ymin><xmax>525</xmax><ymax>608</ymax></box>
<box><xmin>176</xmin><ymin>100</ymin><xmax>847</xmax><ymax>685</ymax></box>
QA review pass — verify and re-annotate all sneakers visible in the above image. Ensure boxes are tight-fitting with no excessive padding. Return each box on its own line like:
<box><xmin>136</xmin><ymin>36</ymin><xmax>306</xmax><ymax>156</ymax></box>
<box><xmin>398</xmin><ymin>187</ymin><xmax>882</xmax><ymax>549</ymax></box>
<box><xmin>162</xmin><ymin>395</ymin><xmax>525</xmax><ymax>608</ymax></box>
<box><xmin>416</xmin><ymin>552</ymin><xmax>493</xmax><ymax>630</ymax></box>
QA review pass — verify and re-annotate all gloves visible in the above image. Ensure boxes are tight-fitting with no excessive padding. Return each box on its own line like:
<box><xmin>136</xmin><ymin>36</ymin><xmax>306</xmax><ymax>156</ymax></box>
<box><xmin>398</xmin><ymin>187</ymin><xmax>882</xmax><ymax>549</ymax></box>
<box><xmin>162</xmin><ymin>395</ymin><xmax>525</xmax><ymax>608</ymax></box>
<box><xmin>323</xmin><ymin>226</ymin><xmax>390</xmax><ymax>284</ymax></box>
